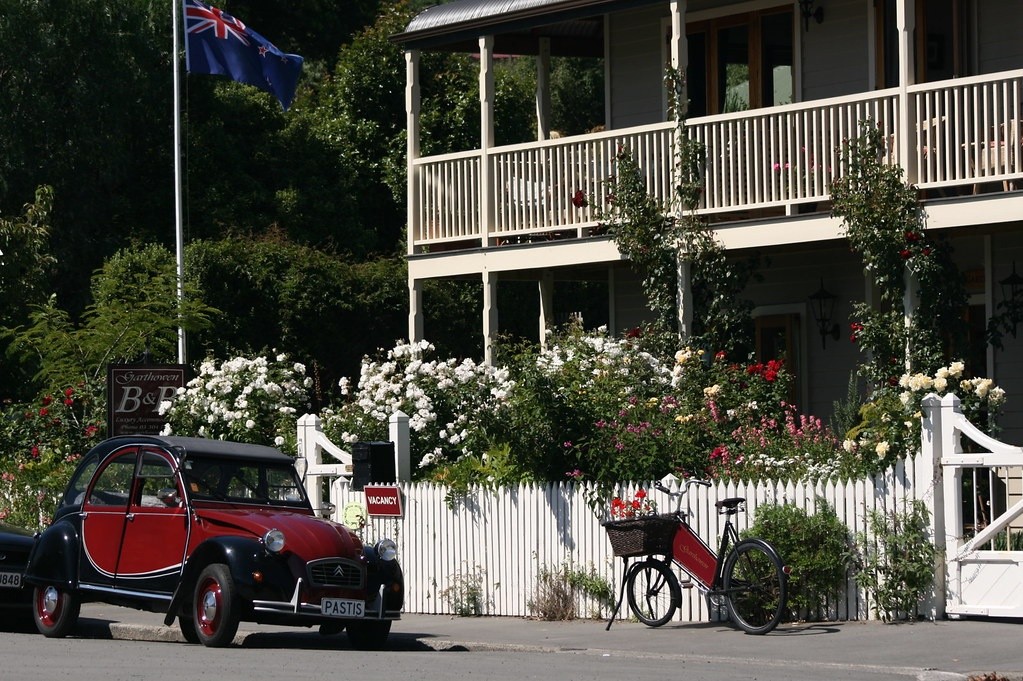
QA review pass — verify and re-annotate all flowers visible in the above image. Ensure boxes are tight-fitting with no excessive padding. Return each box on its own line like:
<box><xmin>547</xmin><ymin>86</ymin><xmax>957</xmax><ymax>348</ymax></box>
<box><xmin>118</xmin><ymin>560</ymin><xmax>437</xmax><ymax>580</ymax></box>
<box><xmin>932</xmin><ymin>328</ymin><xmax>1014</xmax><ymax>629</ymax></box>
<box><xmin>773</xmin><ymin>148</ymin><xmax>832</xmax><ymax>197</ymax></box>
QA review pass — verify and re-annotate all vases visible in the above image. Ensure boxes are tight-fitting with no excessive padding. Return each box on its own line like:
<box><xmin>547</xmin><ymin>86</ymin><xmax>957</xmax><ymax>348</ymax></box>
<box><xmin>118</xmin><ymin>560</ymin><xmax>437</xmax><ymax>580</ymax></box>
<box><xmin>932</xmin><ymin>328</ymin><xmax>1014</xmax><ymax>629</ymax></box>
<box><xmin>798</xmin><ymin>202</ymin><xmax>818</xmax><ymax>214</ymax></box>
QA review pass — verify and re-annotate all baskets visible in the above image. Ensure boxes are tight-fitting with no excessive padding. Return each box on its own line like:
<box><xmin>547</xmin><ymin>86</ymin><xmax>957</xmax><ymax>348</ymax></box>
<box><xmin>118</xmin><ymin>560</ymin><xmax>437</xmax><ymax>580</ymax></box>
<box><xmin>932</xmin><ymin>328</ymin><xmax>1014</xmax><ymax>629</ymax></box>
<box><xmin>603</xmin><ymin>515</ymin><xmax>676</xmax><ymax>557</ymax></box>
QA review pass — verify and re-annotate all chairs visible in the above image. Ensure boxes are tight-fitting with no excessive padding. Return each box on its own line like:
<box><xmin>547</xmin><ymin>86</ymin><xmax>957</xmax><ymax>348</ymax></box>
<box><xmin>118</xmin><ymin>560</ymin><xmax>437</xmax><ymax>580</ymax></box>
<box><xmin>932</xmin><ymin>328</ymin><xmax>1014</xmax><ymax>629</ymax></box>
<box><xmin>881</xmin><ymin>116</ymin><xmax>1023</xmax><ymax>198</ymax></box>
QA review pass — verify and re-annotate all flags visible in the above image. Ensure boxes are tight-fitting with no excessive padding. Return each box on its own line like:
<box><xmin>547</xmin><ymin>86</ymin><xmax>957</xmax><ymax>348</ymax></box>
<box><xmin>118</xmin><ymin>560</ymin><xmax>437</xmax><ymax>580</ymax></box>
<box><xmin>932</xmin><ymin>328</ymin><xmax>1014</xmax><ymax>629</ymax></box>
<box><xmin>183</xmin><ymin>0</ymin><xmax>305</xmax><ymax>113</ymax></box>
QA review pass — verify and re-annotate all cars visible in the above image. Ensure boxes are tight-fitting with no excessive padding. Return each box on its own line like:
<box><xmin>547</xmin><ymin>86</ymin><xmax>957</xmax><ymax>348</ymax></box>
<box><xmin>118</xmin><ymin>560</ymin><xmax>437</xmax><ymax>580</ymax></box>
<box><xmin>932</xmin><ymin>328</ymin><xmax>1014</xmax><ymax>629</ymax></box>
<box><xmin>20</xmin><ymin>432</ymin><xmax>406</xmax><ymax>651</ymax></box>
<box><xmin>0</xmin><ymin>523</ymin><xmax>41</xmax><ymax>604</ymax></box>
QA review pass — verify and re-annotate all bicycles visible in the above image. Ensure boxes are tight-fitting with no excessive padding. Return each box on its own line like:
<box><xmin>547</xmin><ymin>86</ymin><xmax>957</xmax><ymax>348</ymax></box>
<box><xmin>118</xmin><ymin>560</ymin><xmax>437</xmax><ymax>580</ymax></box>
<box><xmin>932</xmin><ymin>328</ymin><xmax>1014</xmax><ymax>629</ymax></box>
<box><xmin>601</xmin><ymin>479</ymin><xmax>791</xmax><ymax>636</ymax></box>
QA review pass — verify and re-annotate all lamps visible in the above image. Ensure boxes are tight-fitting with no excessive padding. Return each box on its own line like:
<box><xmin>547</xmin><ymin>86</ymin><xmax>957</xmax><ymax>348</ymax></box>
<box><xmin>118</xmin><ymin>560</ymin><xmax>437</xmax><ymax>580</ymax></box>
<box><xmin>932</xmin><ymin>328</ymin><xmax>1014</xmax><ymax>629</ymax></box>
<box><xmin>797</xmin><ymin>0</ymin><xmax>823</xmax><ymax>32</ymax></box>
<box><xmin>999</xmin><ymin>261</ymin><xmax>1023</xmax><ymax>339</ymax></box>
<box><xmin>808</xmin><ymin>279</ymin><xmax>840</xmax><ymax>351</ymax></box>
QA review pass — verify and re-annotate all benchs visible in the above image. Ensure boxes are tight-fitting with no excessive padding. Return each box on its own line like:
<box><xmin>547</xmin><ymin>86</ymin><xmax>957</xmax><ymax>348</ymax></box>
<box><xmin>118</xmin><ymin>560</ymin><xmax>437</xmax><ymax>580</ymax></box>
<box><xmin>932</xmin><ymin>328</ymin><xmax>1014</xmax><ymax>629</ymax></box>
<box><xmin>73</xmin><ymin>491</ymin><xmax>180</xmax><ymax>507</ymax></box>
